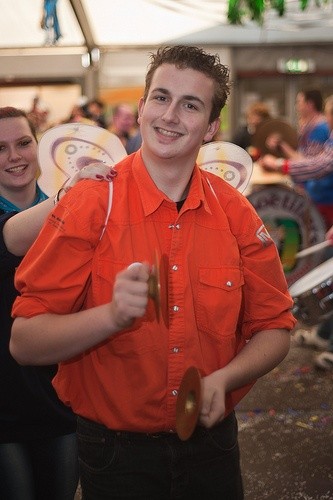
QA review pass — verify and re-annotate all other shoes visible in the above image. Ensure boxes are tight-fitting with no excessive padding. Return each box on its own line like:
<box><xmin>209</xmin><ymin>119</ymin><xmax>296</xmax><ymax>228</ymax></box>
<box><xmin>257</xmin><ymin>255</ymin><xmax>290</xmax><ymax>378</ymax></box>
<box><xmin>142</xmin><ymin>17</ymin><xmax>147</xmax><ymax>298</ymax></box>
<box><xmin>294</xmin><ymin>325</ymin><xmax>333</xmax><ymax>351</ymax></box>
<box><xmin>314</xmin><ymin>352</ymin><xmax>333</xmax><ymax>370</ymax></box>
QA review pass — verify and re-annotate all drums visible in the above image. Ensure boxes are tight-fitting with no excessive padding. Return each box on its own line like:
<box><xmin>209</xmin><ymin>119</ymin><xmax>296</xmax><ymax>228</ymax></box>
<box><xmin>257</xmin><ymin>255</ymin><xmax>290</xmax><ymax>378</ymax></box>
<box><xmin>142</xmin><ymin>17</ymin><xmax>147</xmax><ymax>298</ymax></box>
<box><xmin>242</xmin><ymin>182</ymin><xmax>327</xmax><ymax>319</ymax></box>
<box><xmin>287</xmin><ymin>256</ymin><xmax>333</xmax><ymax>330</ymax></box>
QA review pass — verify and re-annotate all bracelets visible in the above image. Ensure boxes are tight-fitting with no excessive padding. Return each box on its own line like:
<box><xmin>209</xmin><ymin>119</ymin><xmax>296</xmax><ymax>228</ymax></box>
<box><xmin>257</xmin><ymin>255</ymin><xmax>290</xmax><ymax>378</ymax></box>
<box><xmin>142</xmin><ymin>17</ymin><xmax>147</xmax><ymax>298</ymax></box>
<box><xmin>54</xmin><ymin>188</ymin><xmax>63</xmax><ymax>204</ymax></box>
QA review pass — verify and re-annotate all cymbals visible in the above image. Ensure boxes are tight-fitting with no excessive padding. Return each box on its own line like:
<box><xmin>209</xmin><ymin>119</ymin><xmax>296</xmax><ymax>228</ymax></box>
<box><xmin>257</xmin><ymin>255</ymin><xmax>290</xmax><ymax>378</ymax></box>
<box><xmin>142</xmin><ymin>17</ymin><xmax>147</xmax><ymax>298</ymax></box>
<box><xmin>254</xmin><ymin>119</ymin><xmax>299</xmax><ymax>158</ymax></box>
<box><xmin>174</xmin><ymin>365</ymin><xmax>202</xmax><ymax>442</ymax></box>
<box><xmin>147</xmin><ymin>253</ymin><xmax>162</xmax><ymax>325</ymax></box>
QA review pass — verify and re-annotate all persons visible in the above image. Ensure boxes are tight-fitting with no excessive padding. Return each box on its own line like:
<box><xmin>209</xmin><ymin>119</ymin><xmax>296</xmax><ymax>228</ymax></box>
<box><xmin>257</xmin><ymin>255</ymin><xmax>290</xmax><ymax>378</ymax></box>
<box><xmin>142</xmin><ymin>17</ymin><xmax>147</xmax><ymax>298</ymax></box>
<box><xmin>261</xmin><ymin>95</ymin><xmax>333</xmax><ymax>181</ymax></box>
<box><xmin>110</xmin><ymin>103</ymin><xmax>133</xmax><ymax>145</ymax></box>
<box><xmin>10</xmin><ymin>40</ymin><xmax>297</xmax><ymax>500</ymax></box>
<box><xmin>295</xmin><ymin>228</ymin><xmax>333</xmax><ymax>371</ymax></box>
<box><xmin>267</xmin><ymin>90</ymin><xmax>333</xmax><ymax>229</ymax></box>
<box><xmin>247</xmin><ymin>102</ymin><xmax>297</xmax><ymax>159</ymax></box>
<box><xmin>68</xmin><ymin>100</ymin><xmax>107</xmax><ymax>128</ymax></box>
<box><xmin>0</xmin><ymin>106</ymin><xmax>118</xmax><ymax>500</ymax></box>
<box><xmin>125</xmin><ymin>106</ymin><xmax>142</xmax><ymax>155</ymax></box>
